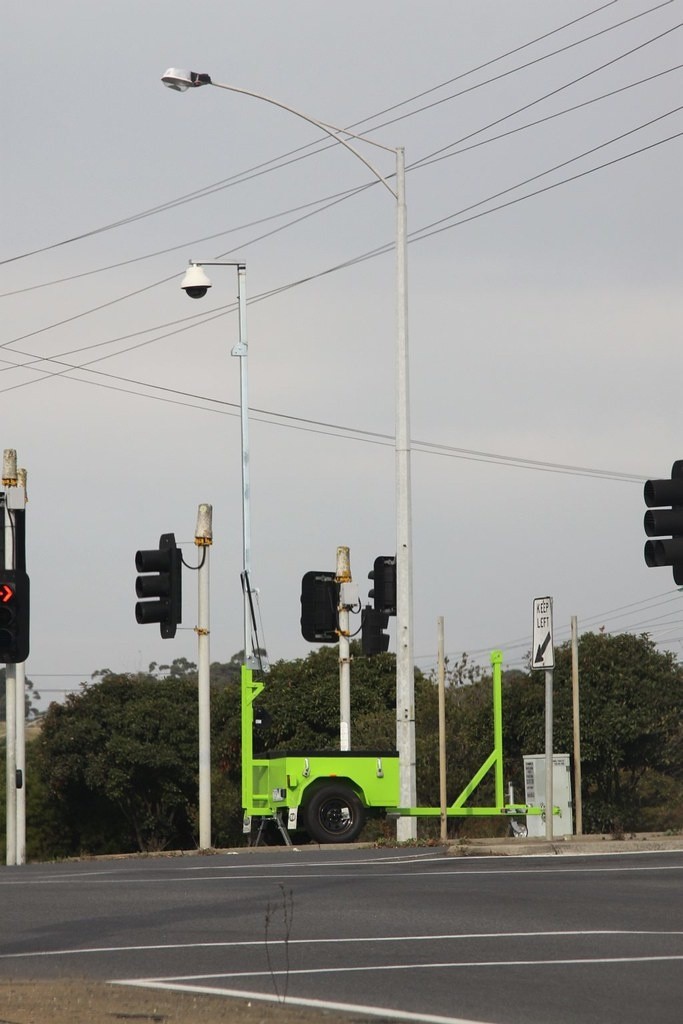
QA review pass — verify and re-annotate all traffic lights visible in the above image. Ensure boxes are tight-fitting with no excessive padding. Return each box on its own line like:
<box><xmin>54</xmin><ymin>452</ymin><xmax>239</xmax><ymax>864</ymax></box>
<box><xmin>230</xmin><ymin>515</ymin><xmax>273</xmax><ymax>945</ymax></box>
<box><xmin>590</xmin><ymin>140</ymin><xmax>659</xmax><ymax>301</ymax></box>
<box><xmin>641</xmin><ymin>460</ymin><xmax>683</xmax><ymax>589</ymax></box>
<box><xmin>360</xmin><ymin>607</ymin><xmax>390</xmax><ymax>655</ymax></box>
<box><xmin>0</xmin><ymin>571</ymin><xmax>32</xmax><ymax>663</ymax></box>
<box><xmin>367</xmin><ymin>556</ymin><xmax>397</xmax><ymax>615</ymax></box>
<box><xmin>135</xmin><ymin>533</ymin><xmax>184</xmax><ymax>639</ymax></box>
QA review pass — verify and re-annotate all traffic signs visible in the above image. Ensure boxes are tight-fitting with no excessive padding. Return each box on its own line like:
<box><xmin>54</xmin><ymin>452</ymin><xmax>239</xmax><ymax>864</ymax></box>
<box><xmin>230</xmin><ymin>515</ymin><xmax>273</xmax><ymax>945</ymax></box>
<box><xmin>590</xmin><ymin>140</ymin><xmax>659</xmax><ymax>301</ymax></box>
<box><xmin>528</xmin><ymin>594</ymin><xmax>555</xmax><ymax>673</ymax></box>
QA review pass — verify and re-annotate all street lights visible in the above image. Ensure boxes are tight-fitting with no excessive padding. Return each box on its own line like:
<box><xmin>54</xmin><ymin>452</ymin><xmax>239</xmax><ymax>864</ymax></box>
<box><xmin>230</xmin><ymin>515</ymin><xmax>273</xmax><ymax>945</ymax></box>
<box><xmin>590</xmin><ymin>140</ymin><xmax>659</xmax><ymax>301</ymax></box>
<box><xmin>182</xmin><ymin>256</ymin><xmax>257</xmax><ymax>837</ymax></box>
<box><xmin>159</xmin><ymin>66</ymin><xmax>418</xmax><ymax>851</ymax></box>
